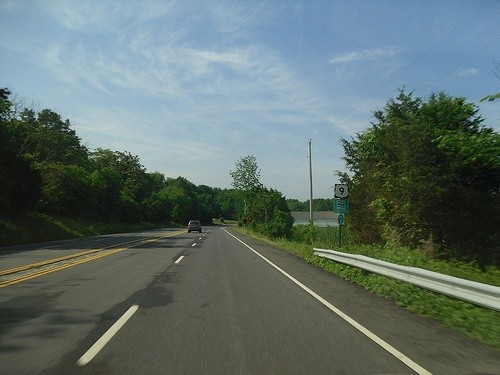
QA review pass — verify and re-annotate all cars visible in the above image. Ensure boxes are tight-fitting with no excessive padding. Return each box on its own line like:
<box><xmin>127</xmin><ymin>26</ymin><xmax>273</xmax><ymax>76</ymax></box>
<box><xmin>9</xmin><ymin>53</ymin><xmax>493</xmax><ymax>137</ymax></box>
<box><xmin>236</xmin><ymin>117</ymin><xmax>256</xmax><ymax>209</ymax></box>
<box><xmin>187</xmin><ymin>220</ymin><xmax>202</xmax><ymax>234</ymax></box>
